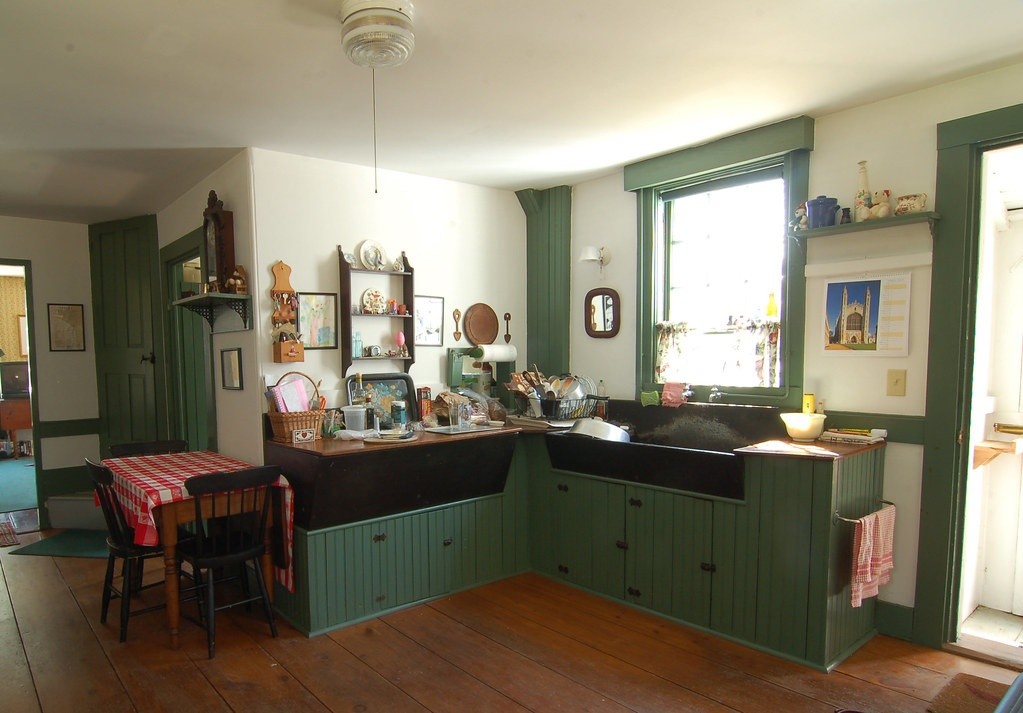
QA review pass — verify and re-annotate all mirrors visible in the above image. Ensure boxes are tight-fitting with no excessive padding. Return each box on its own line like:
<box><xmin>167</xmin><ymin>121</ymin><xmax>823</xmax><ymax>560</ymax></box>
<box><xmin>584</xmin><ymin>287</ymin><xmax>620</xmax><ymax>338</ymax></box>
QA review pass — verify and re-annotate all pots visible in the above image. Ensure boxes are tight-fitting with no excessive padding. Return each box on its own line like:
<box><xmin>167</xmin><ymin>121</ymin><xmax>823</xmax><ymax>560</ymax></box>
<box><xmin>559</xmin><ymin>378</ymin><xmax>611</xmax><ymax>413</ymax></box>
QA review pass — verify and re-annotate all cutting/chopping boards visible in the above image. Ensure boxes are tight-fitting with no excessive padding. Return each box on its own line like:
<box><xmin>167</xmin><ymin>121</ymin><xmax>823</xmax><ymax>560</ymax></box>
<box><xmin>363</xmin><ymin>437</ymin><xmax>418</xmax><ymax>443</ymax></box>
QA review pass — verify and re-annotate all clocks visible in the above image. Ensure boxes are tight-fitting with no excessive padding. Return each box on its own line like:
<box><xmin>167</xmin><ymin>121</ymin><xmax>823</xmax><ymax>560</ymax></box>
<box><xmin>203</xmin><ymin>189</ymin><xmax>234</xmax><ymax>291</ymax></box>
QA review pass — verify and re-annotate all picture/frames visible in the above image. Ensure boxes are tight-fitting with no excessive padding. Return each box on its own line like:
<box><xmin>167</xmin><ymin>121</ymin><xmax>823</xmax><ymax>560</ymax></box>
<box><xmin>47</xmin><ymin>303</ymin><xmax>86</xmax><ymax>351</ymax></box>
<box><xmin>221</xmin><ymin>347</ymin><xmax>243</xmax><ymax>391</ymax></box>
<box><xmin>296</xmin><ymin>292</ymin><xmax>338</xmax><ymax>350</ymax></box>
<box><xmin>413</xmin><ymin>294</ymin><xmax>445</xmax><ymax>346</ymax></box>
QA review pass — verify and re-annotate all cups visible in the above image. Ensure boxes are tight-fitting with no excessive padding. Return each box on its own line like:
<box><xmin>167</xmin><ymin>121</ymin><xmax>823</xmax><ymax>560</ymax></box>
<box><xmin>198</xmin><ymin>283</ymin><xmax>210</xmax><ymax>294</ymax></box>
<box><xmin>391</xmin><ymin>400</ymin><xmax>406</xmax><ymax>430</ymax></box>
<box><xmin>351</xmin><ymin>304</ymin><xmax>360</xmax><ymax>314</ymax></box>
<box><xmin>399</xmin><ymin>304</ymin><xmax>406</xmax><ymax>314</ymax></box>
<box><xmin>449</xmin><ymin>402</ymin><xmax>463</xmax><ymax>429</ymax></box>
<box><xmin>324</xmin><ymin>419</ymin><xmax>340</xmax><ymax>438</ymax></box>
<box><xmin>458</xmin><ymin>405</ymin><xmax>472</xmax><ymax>428</ymax></box>
<box><xmin>342</xmin><ymin>406</ymin><xmax>366</xmax><ymax>430</ymax></box>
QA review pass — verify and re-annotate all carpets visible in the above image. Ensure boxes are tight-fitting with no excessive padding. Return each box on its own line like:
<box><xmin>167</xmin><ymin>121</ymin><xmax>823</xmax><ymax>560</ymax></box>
<box><xmin>0</xmin><ymin>522</ymin><xmax>20</xmax><ymax>547</ymax></box>
<box><xmin>9</xmin><ymin>528</ymin><xmax>120</xmax><ymax>559</ymax></box>
<box><xmin>926</xmin><ymin>672</ymin><xmax>1010</xmax><ymax>713</ymax></box>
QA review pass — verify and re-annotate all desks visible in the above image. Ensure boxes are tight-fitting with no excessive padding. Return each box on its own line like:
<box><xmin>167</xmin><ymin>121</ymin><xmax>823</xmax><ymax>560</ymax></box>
<box><xmin>0</xmin><ymin>398</ymin><xmax>32</xmax><ymax>460</ymax></box>
<box><xmin>95</xmin><ymin>450</ymin><xmax>294</xmax><ymax>635</ymax></box>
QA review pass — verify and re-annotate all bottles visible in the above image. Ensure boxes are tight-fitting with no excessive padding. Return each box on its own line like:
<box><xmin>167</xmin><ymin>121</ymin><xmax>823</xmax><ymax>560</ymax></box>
<box><xmin>817</xmin><ymin>402</ymin><xmax>826</xmax><ymax>417</ymax></box>
<box><xmin>351</xmin><ymin>372</ymin><xmax>366</xmax><ymax>406</ymax></box>
<box><xmin>365</xmin><ymin>393</ymin><xmax>374</xmax><ymax>431</ymax></box>
<box><xmin>392</xmin><ymin>300</ymin><xmax>398</xmax><ymax>315</ymax></box>
<box><xmin>279</xmin><ymin>332</ymin><xmax>287</xmax><ymax>342</ymax></box>
<box><xmin>353</xmin><ymin>332</ymin><xmax>363</xmax><ymax>357</ymax></box>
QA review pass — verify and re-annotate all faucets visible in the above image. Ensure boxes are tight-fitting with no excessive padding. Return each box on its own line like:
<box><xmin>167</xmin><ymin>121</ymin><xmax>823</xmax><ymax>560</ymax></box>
<box><xmin>708</xmin><ymin>385</ymin><xmax>722</xmax><ymax>404</ymax></box>
<box><xmin>681</xmin><ymin>383</ymin><xmax>695</xmax><ymax>401</ymax></box>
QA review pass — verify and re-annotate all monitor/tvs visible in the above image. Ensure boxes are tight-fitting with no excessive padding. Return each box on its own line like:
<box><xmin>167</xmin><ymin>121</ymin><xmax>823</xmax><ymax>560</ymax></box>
<box><xmin>0</xmin><ymin>361</ymin><xmax>30</xmax><ymax>400</ymax></box>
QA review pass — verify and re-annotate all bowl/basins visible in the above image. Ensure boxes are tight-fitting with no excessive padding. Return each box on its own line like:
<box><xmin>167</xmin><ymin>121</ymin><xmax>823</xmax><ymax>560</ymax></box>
<box><xmin>566</xmin><ymin>418</ymin><xmax>631</xmax><ymax>444</ymax></box>
<box><xmin>529</xmin><ymin>393</ymin><xmax>561</xmax><ymax>417</ymax></box>
<box><xmin>489</xmin><ymin>421</ymin><xmax>506</xmax><ymax>426</ymax></box>
<box><xmin>471</xmin><ymin>414</ymin><xmax>487</xmax><ymax>421</ymax></box>
<box><xmin>779</xmin><ymin>412</ymin><xmax>829</xmax><ymax>442</ymax></box>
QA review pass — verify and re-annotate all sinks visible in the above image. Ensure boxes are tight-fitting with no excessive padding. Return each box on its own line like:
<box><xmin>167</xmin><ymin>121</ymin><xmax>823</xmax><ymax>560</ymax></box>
<box><xmin>547</xmin><ymin>424</ymin><xmax>764</xmax><ymax>456</ymax></box>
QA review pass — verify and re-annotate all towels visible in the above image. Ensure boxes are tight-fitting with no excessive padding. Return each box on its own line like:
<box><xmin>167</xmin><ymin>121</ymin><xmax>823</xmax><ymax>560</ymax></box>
<box><xmin>853</xmin><ymin>504</ymin><xmax>895</xmax><ymax>609</ymax></box>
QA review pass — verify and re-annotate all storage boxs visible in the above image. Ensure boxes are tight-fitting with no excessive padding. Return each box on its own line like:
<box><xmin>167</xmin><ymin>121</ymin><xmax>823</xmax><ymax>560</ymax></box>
<box><xmin>44</xmin><ymin>491</ymin><xmax>108</xmax><ymax>531</ymax></box>
<box><xmin>340</xmin><ymin>405</ymin><xmax>365</xmax><ymax>432</ymax></box>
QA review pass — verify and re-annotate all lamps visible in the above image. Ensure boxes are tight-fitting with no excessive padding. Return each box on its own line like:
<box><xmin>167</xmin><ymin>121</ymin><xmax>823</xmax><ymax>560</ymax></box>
<box><xmin>340</xmin><ymin>0</ymin><xmax>414</xmax><ymax>69</ymax></box>
<box><xmin>579</xmin><ymin>245</ymin><xmax>611</xmax><ymax>266</ymax></box>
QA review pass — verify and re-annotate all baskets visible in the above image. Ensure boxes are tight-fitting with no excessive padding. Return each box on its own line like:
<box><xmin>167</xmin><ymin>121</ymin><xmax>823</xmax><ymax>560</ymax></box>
<box><xmin>268</xmin><ymin>372</ymin><xmax>325</xmax><ymax>443</ymax></box>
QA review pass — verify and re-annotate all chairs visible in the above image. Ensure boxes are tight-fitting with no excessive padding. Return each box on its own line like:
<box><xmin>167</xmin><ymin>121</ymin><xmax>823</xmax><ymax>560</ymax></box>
<box><xmin>173</xmin><ymin>463</ymin><xmax>280</xmax><ymax>658</ymax></box>
<box><xmin>84</xmin><ymin>458</ymin><xmax>207</xmax><ymax>643</ymax></box>
<box><xmin>107</xmin><ymin>440</ymin><xmax>189</xmax><ymax>459</ymax></box>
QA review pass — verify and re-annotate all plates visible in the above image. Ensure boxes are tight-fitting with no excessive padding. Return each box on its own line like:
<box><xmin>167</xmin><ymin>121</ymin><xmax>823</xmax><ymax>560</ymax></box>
<box><xmin>380</xmin><ymin>430</ymin><xmax>408</xmax><ymax>439</ymax></box>
<box><xmin>360</xmin><ymin>240</ymin><xmax>387</xmax><ymax>270</ymax></box>
<box><xmin>363</xmin><ymin>288</ymin><xmax>387</xmax><ymax>311</ymax></box>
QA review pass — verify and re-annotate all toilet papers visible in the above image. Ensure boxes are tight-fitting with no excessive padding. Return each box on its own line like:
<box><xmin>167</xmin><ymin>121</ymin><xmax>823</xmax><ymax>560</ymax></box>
<box><xmin>476</xmin><ymin>344</ymin><xmax>518</xmax><ymax>363</ymax></box>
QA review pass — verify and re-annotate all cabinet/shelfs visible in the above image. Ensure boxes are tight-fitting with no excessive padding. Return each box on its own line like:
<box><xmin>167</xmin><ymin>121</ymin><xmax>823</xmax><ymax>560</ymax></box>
<box><xmin>264</xmin><ymin>440</ymin><xmax>515</xmax><ymax>639</ymax></box>
<box><xmin>516</xmin><ymin>432</ymin><xmax>887</xmax><ymax>672</ymax></box>
